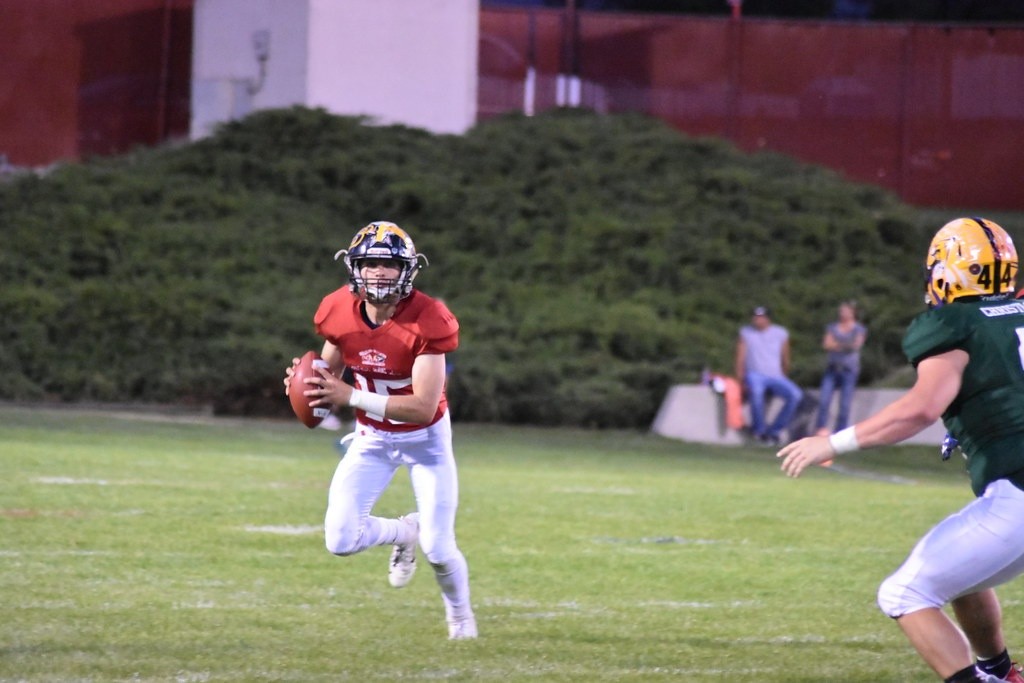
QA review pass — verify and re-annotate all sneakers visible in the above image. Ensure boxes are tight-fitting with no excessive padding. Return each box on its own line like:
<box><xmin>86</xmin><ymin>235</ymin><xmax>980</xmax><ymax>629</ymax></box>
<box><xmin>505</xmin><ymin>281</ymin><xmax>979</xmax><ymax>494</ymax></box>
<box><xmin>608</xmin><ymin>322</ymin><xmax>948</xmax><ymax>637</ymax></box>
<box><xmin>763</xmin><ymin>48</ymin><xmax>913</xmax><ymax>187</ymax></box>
<box><xmin>446</xmin><ymin>606</ymin><xmax>477</xmax><ymax>639</ymax></box>
<box><xmin>388</xmin><ymin>512</ymin><xmax>423</xmax><ymax>588</ymax></box>
<box><xmin>973</xmin><ymin>664</ymin><xmax>1024</xmax><ymax>683</ymax></box>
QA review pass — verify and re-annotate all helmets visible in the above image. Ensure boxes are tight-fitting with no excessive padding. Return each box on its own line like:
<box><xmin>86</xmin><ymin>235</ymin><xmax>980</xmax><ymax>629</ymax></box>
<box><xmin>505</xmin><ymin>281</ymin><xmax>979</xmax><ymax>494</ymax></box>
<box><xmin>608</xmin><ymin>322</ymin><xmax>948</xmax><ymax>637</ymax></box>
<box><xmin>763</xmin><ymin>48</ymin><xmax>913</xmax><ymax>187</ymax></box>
<box><xmin>923</xmin><ymin>216</ymin><xmax>1020</xmax><ymax>305</ymax></box>
<box><xmin>346</xmin><ymin>221</ymin><xmax>417</xmax><ymax>304</ymax></box>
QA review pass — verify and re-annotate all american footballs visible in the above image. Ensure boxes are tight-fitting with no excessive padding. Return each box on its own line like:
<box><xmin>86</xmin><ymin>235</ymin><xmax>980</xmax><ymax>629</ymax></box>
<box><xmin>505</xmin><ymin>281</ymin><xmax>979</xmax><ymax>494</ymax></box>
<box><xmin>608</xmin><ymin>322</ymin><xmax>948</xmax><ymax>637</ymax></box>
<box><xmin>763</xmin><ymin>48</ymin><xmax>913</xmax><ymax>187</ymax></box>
<box><xmin>288</xmin><ymin>351</ymin><xmax>333</xmax><ymax>429</ymax></box>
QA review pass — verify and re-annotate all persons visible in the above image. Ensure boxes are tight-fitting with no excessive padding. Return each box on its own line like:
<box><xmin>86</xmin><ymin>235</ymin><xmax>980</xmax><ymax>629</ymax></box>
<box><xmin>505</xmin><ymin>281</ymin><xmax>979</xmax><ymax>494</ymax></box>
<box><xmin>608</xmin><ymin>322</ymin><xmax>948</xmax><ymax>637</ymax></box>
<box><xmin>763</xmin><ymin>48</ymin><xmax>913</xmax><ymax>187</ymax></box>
<box><xmin>733</xmin><ymin>303</ymin><xmax>803</xmax><ymax>447</ymax></box>
<box><xmin>282</xmin><ymin>220</ymin><xmax>480</xmax><ymax>644</ymax></box>
<box><xmin>811</xmin><ymin>297</ymin><xmax>868</xmax><ymax>434</ymax></box>
<box><xmin>771</xmin><ymin>212</ymin><xmax>1024</xmax><ymax>683</ymax></box>
